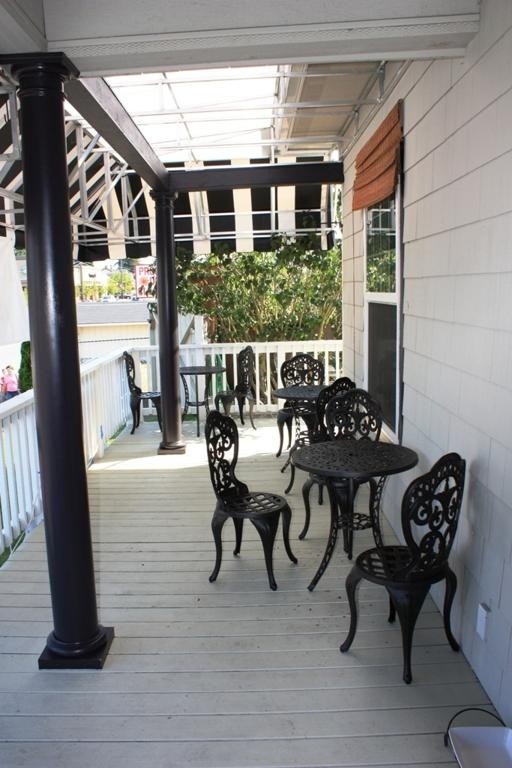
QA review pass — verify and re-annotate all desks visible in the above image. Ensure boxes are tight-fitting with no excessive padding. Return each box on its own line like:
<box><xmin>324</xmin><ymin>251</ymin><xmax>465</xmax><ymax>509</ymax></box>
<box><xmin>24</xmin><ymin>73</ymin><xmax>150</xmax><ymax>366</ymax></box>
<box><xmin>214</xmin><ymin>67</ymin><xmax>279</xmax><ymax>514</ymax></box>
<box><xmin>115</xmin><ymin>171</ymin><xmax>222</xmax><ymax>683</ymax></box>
<box><xmin>180</xmin><ymin>366</ymin><xmax>227</xmax><ymax>438</ymax></box>
<box><xmin>290</xmin><ymin>440</ymin><xmax>419</xmax><ymax>591</ymax></box>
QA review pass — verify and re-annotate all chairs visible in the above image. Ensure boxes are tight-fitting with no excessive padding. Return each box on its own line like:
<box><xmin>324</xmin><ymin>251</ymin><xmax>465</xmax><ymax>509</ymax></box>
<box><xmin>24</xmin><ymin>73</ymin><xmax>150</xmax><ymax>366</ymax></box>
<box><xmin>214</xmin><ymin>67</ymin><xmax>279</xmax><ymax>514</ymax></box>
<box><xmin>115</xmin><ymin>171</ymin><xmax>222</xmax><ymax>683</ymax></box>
<box><xmin>205</xmin><ymin>409</ymin><xmax>297</xmax><ymax>591</ymax></box>
<box><xmin>214</xmin><ymin>346</ymin><xmax>256</xmax><ymax>430</ymax></box>
<box><xmin>340</xmin><ymin>452</ymin><xmax>466</xmax><ymax>685</ymax></box>
<box><xmin>277</xmin><ymin>354</ymin><xmax>383</xmax><ymax>552</ymax></box>
<box><xmin>124</xmin><ymin>351</ymin><xmax>162</xmax><ymax>434</ymax></box>
